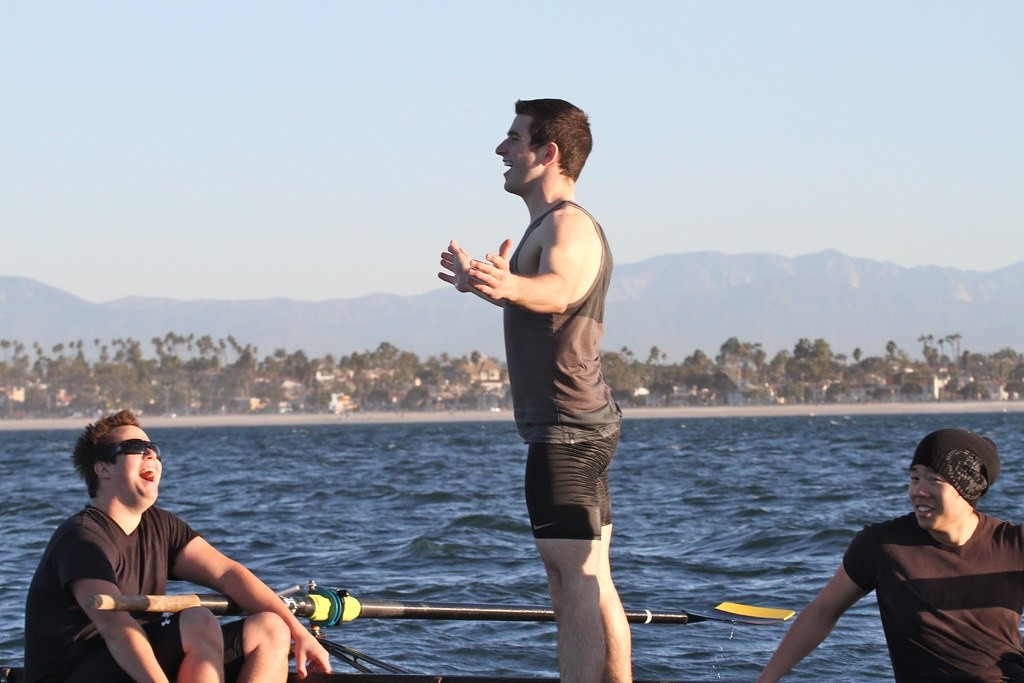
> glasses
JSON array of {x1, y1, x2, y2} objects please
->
[{"x1": 104, "y1": 438, "x2": 161, "y2": 463}]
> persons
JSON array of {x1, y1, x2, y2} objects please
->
[
  {"x1": 754, "y1": 427, "x2": 1024, "y2": 683},
  {"x1": 22, "y1": 409, "x2": 333, "y2": 683},
  {"x1": 439, "y1": 97, "x2": 635, "y2": 683}
]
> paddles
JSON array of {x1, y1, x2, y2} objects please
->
[{"x1": 91, "y1": 581, "x2": 796, "y2": 674}]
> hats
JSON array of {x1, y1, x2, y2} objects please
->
[{"x1": 912, "y1": 427, "x2": 1000, "y2": 507}]
